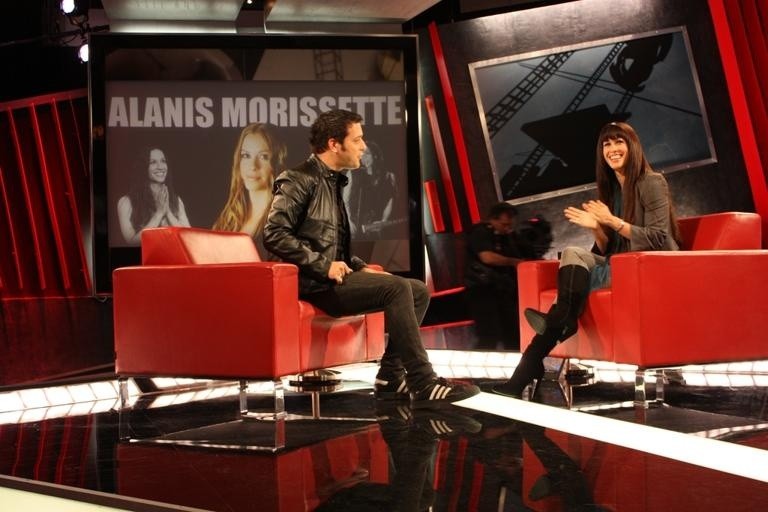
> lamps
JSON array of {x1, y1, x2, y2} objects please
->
[
  {"x1": 110, "y1": 225, "x2": 388, "y2": 455},
  {"x1": 514, "y1": 211, "x2": 766, "y2": 410}
]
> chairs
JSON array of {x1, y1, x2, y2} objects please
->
[
  {"x1": 412, "y1": 377, "x2": 481, "y2": 409},
  {"x1": 374, "y1": 399, "x2": 414, "y2": 423},
  {"x1": 413, "y1": 409, "x2": 482, "y2": 443},
  {"x1": 374, "y1": 372, "x2": 412, "y2": 400}
]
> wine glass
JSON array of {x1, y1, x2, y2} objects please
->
[{"x1": 339, "y1": 273, "x2": 343, "y2": 277}]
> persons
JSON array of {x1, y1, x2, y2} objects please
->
[
  {"x1": 117, "y1": 144, "x2": 191, "y2": 246},
  {"x1": 341, "y1": 140, "x2": 399, "y2": 241},
  {"x1": 209, "y1": 123, "x2": 302, "y2": 262},
  {"x1": 491, "y1": 123, "x2": 680, "y2": 400},
  {"x1": 463, "y1": 203, "x2": 525, "y2": 349},
  {"x1": 263, "y1": 109, "x2": 481, "y2": 409}
]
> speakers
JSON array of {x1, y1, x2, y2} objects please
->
[
  {"x1": 524, "y1": 264, "x2": 590, "y2": 343},
  {"x1": 491, "y1": 303, "x2": 557, "y2": 400}
]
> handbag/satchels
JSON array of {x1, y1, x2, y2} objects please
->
[{"x1": 616, "y1": 218, "x2": 625, "y2": 232}]
